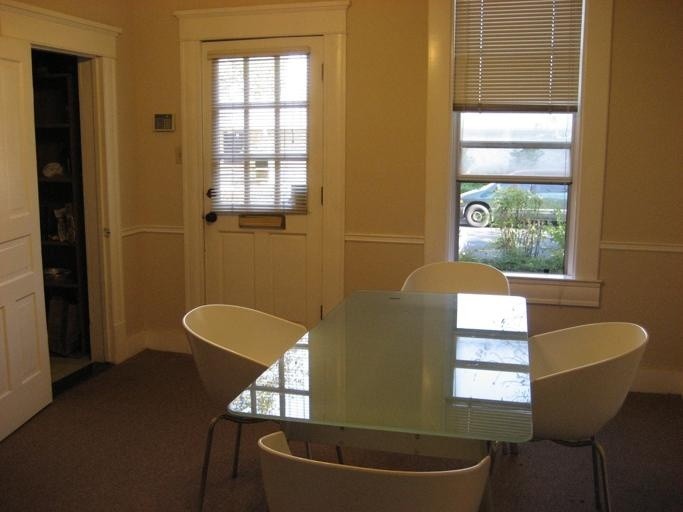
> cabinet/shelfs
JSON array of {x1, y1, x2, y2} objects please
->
[{"x1": 33, "y1": 72, "x2": 88, "y2": 358}]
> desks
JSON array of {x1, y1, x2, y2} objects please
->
[{"x1": 224, "y1": 286, "x2": 538, "y2": 452}]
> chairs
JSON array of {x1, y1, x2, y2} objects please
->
[
  {"x1": 180, "y1": 302, "x2": 347, "y2": 512},
  {"x1": 500, "y1": 320, "x2": 651, "y2": 512},
  {"x1": 391, "y1": 258, "x2": 515, "y2": 440},
  {"x1": 254, "y1": 429, "x2": 495, "y2": 511}
]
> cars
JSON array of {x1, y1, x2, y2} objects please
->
[{"x1": 460, "y1": 169, "x2": 568, "y2": 227}]
